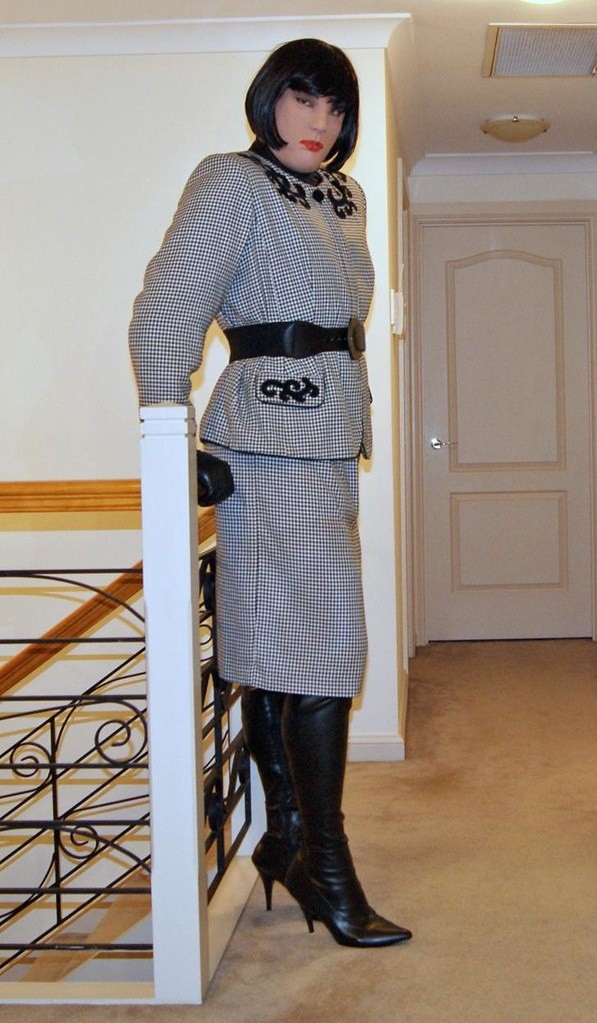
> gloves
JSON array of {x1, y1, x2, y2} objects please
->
[{"x1": 195, "y1": 450, "x2": 236, "y2": 507}]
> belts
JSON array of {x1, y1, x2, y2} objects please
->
[{"x1": 223, "y1": 317, "x2": 366, "y2": 363}]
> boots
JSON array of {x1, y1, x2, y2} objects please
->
[
  {"x1": 288, "y1": 694, "x2": 412, "y2": 948},
  {"x1": 240, "y1": 686, "x2": 304, "y2": 912}
]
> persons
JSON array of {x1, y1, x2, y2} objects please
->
[{"x1": 127, "y1": 40, "x2": 410, "y2": 948}]
